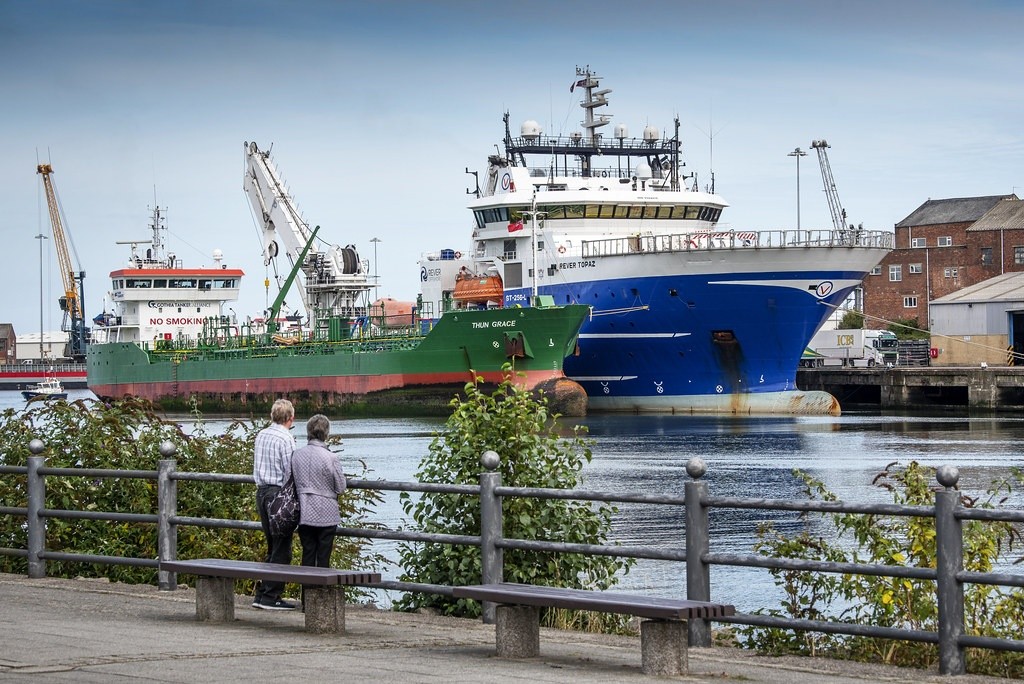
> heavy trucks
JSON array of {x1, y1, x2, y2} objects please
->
[{"x1": 799, "y1": 330, "x2": 900, "y2": 368}]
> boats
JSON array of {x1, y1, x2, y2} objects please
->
[
  {"x1": 453, "y1": 271, "x2": 504, "y2": 304},
  {"x1": 86, "y1": 183, "x2": 595, "y2": 421},
  {"x1": 21, "y1": 373, "x2": 68, "y2": 402},
  {"x1": 419, "y1": 63, "x2": 895, "y2": 416}
]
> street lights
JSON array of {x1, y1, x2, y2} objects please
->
[
  {"x1": 786, "y1": 147, "x2": 809, "y2": 246},
  {"x1": 35, "y1": 234, "x2": 48, "y2": 364},
  {"x1": 368, "y1": 237, "x2": 382, "y2": 302}
]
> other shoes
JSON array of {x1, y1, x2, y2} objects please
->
[
  {"x1": 252, "y1": 595, "x2": 261, "y2": 608},
  {"x1": 259, "y1": 595, "x2": 295, "y2": 611},
  {"x1": 302, "y1": 604, "x2": 305, "y2": 613}
]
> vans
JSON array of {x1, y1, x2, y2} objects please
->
[{"x1": 22, "y1": 360, "x2": 33, "y2": 364}]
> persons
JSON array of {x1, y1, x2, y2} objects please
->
[
  {"x1": 251, "y1": 398, "x2": 297, "y2": 610},
  {"x1": 291, "y1": 414, "x2": 346, "y2": 613}
]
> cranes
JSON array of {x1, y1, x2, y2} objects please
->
[
  {"x1": 35, "y1": 144, "x2": 83, "y2": 323},
  {"x1": 243, "y1": 141, "x2": 381, "y2": 340}
]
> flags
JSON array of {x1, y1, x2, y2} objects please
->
[{"x1": 507, "y1": 220, "x2": 523, "y2": 232}]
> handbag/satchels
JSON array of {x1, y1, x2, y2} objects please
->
[{"x1": 265, "y1": 451, "x2": 300, "y2": 538}]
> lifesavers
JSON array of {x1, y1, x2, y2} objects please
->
[
  {"x1": 602, "y1": 170, "x2": 607, "y2": 177},
  {"x1": 455, "y1": 252, "x2": 461, "y2": 259},
  {"x1": 558, "y1": 245, "x2": 566, "y2": 254}
]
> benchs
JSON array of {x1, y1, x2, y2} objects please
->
[
  {"x1": 453, "y1": 581, "x2": 736, "y2": 678},
  {"x1": 160, "y1": 559, "x2": 382, "y2": 635}
]
